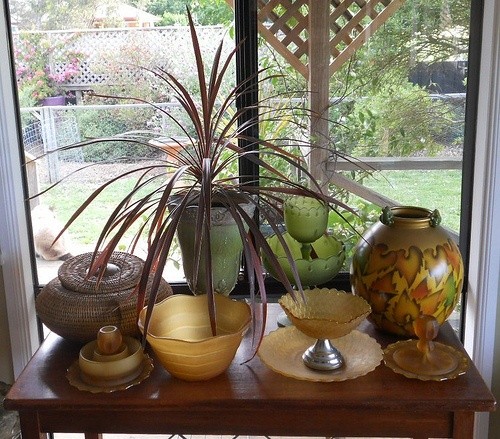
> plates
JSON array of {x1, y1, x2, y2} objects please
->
[
  {"x1": 384, "y1": 339, "x2": 469, "y2": 381},
  {"x1": 66, "y1": 354, "x2": 154, "y2": 394},
  {"x1": 257, "y1": 326, "x2": 382, "y2": 383}
]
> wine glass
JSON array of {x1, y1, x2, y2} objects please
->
[{"x1": 283, "y1": 196, "x2": 330, "y2": 258}]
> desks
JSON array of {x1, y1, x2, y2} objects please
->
[
  {"x1": 149, "y1": 135, "x2": 196, "y2": 173},
  {"x1": 3, "y1": 300, "x2": 497, "y2": 439}
]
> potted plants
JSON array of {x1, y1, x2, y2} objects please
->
[{"x1": 19, "y1": 7, "x2": 393, "y2": 364}]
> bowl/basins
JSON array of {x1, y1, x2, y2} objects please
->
[
  {"x1": 262, "y1": 230, "x2": 346, "y2": 285},
  {"x1": 78, "y1": 335, "x2": 144, "y2": 382},
  {"x1": 93, "y1": 344, "x2": 128, "y2": 362},
  {"x1": 279, "y1": 288, "x2": 372, "y2": 370},
  {"x1": 137, "y1": 292, "x2": 252, "y2": 381}
]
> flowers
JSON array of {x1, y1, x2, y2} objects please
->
[{"x1": 13, "y1": 46, "x2": 74, "y2": 99}]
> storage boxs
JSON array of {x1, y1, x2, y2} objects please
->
[{"x1": 36, "y1": 250, "x2": 173, "y2": 346}]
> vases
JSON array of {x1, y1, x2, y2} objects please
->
[{"x1": 42, "y1": 96, "x2": 68, "y2": 123}]
